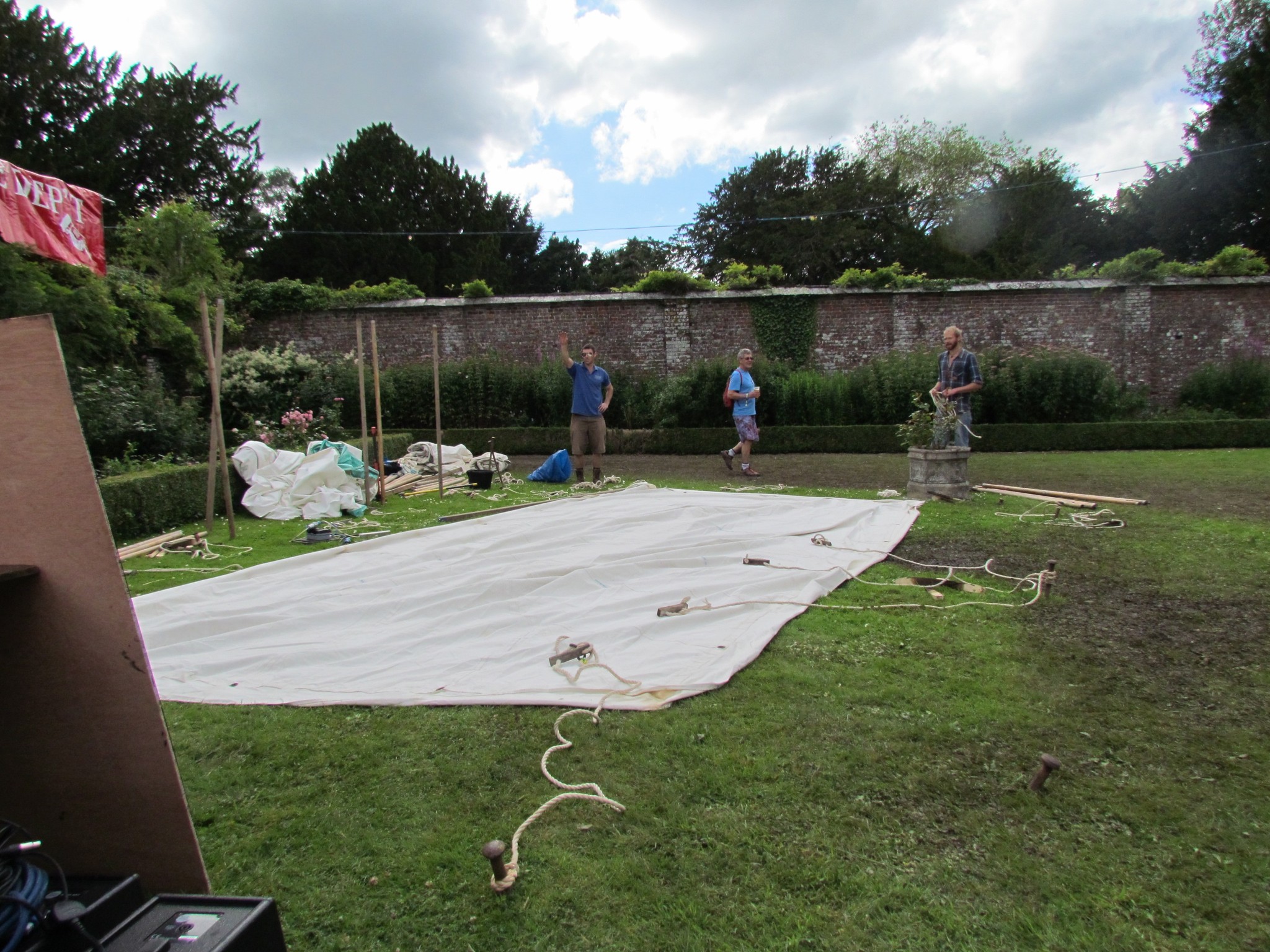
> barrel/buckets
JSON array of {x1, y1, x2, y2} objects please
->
[{"x1": 466, "y1": 470, "x2": 493, "y2": 489}]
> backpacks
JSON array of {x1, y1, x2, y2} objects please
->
[{"x1": 723, "y1": 369, "x2": 742, "y2": 408}]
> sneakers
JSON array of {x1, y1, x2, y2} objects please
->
[
  {"x1": 721, "y1": 450, "x2": 734, "y2": 471},
  {"x1": 741, "y1": 467, "x2": 760, "y2": 477}
]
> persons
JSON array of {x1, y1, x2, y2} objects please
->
[
  {"x1": 721, "y1": 348, "x2": 760, "y2": 476},
  {"x1": 929, "y1": 326, "x2": 982, "y2": 447},
  {"x1": 558, "y1": 331, "x2": 613, "y2": 488}
]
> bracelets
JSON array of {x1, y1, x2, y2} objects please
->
[{"x1": 746, "y1": 394, "x2": 748, "y2": 398}]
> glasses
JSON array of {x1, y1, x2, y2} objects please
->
[
  {"x1": 581, "y1": 352, "x2": 593, "y2": 357},
  {"x1": 742, "y1": 357, "x2": 754, "y2": 361}
]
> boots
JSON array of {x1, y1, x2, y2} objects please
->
[
  {"x1": 592, "y1": 468, "x2": 601, "y2": 486},
  {"x1": 575, "y1": 468, "x2": 585, "y2": 485}
]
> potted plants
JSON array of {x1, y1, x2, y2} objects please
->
[{"x1": 896, "y1": 390, "x2": 971, "y2": 499}]
